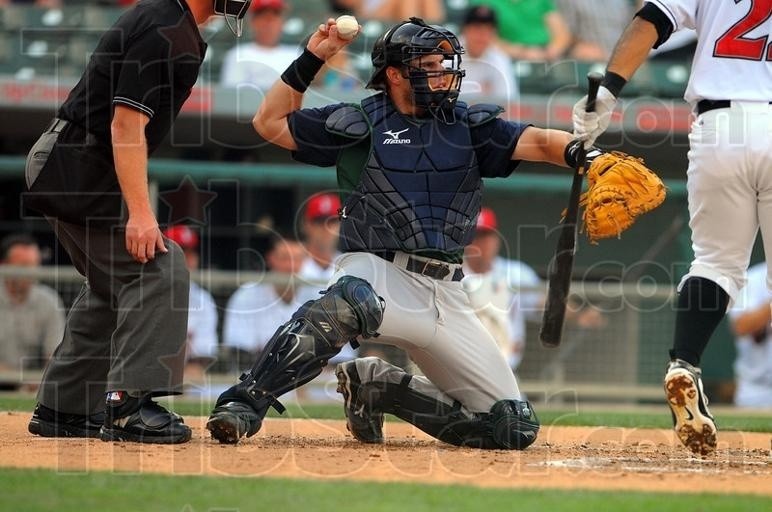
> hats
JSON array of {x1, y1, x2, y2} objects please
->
[
  {"x1": 478, "y1": 208, "x2": 497, "y2": 232},
  {"x1": 249, "y1": 0, "x2": 283, "y2": 13},
  {"x1": 306, "y1": 195, "x2": 341, "y2": 219},
  {"x1": 162, "y1": 224, "x2": 198, "y2": 246},
  {"x1": 466, "y1": 7, "x2": 495, "y2": 24}
]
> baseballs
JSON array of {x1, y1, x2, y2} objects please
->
[{"x1": 334, "y1": 15, "x2": 360, "y2": 40}]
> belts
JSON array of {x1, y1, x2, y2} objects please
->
[
  {"x1": 696, "y1": 100, "x2": 730, "y2": 114},
  {"x1": 377, "y1": 251, "x2": 464, "y2": 281},
  {"x1": 47, "y1": 118, "x2": 68, "y2": 133}
]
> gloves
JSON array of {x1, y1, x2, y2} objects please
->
[{"x1": 572, "y1": 70, "x2": 627, "y2": 150}]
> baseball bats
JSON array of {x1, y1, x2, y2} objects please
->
[{"x1": 541, "y1": 72, "x2": 604, "y2": 348}]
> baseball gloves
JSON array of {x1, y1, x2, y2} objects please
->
[{"x1": 586, "y1": 153, "x2": 667, "y2": 238}]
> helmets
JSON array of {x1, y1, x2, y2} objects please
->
[
  {"x1": 213, "y1": 0, "x2": 252, "y2": 38},
  {"x1": 365, "y1": 16, "x2": 466, "y2": 125}
]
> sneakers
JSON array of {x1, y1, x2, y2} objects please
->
[
  {"x1": 28, "y1": 402, "x2": 108, "y2": 438},
  {"x1": 101, "y1": 397, "x2": 191, "y2": 444},
  {"x1": 336, "y1": 356, "x2": 384, "y2": 443},
  {"x1": 663, "y1": 358, "x2": 718, "y2": 454},
  {"x1": 206, "y1": 401, "x2": 261, "y2": 443}
]
